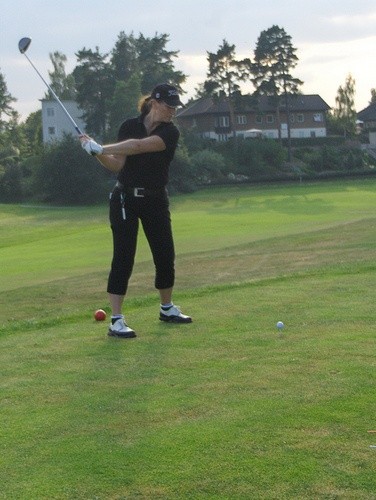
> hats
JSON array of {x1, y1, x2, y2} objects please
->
[{"x1": 151, "y1": 84, "x2": 185, "y2": 109}]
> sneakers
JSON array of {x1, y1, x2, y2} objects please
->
[
  {"x1": 108, "y1": 317, "x2": 136, "y2": 338},
  {"x1": 159, "y1": 304, "x2": 192, "y2": 323}
]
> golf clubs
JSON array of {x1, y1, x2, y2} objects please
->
[{"x1": 19, "y1": 37, "x2": 96, "y2": 156}]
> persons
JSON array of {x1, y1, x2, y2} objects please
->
[{"x1": 78, "y1": 84, "x2": 192, "y2": 336}]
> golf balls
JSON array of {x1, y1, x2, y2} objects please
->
[{"x1": 276, "y1": 321, "x2": 284, "y2": 327}]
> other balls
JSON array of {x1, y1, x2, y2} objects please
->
[{"x1": 95, "y1": 310, "x2": 106, "y2": 320}]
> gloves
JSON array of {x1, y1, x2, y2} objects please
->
[{"x1": 80, "y1": 139, "x2": 103, "y2": 156}]
